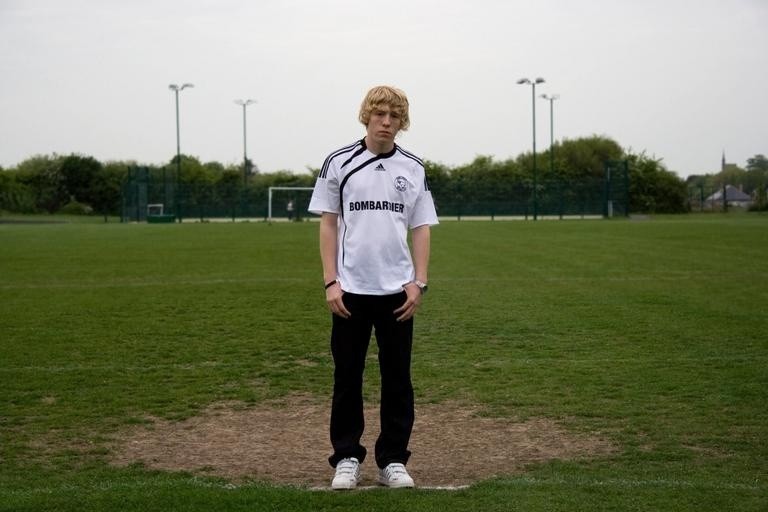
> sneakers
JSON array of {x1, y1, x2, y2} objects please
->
[
  {"x1": 332, "y1": 457, "x2": 361, "y2": 489},
  {"x1": 377, "y1": 463, "x2": 415, "y2": 488}
]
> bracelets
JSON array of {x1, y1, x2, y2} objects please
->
[{"x1": 324, "y1": 279, "x2": 338, "y2": 289}]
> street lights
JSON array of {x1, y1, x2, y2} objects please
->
[
  {"x1": 516, "y1": 77, "x2": 562, "y2": 221},
  {"x1": 234, "y1": 97, "x2": 257, "y2": 216},
  {"x1": 168, "y1": 82, "x2": 195, "y2": 224}
]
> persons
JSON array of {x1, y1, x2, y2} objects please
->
[
  {"x1": 286, "y1": 199, "x2": 293, "y2": 220},
  {"x1": 307, "y1": 86, "x2": 440, "y2": 490}
]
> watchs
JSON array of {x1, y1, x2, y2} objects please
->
[{"x1": 414, "y1": 280, "x2": 428, "y2": 293}]
